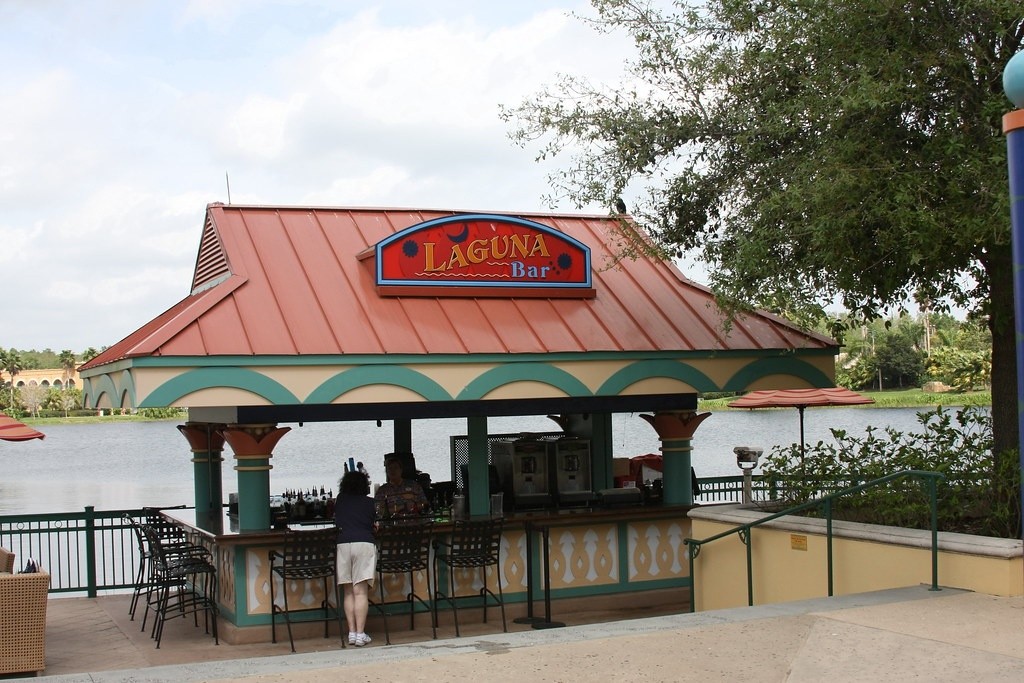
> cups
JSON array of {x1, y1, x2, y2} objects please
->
[{"x1": 492, "y1": 495, "x2": 503, "y2": 514}]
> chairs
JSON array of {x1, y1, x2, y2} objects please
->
[
  {"x1": 125, "y1": 511, "x2": 221, "y2": 650},
  {"x1": 268, "y1": 526, "x2": 345, "y2": 653},
  {"x1": 0, "y1": 547, "x2": 50, "y2": 679},
  {"x1": 432, "y1": 516, "x2": 507, "y2": 637},
  {"x1": 368, "y1": 520, "x2": 437, "y2": 646}
]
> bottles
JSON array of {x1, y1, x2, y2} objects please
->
[
  {"x1": 392, "y1": 503, "x2": 399, "y2": 525},
  {"x1": 420, "y1": 504, "x2": 426, "y2": 521},
  {"x1": 428, "y1": 504, "x2": 433, "y2": 521},
  {"x1": 403, "y1": 502, "x2": 409, "y2": 524},
  {"x1": 435, "y1": 492, "x2": 442, "y2": 522},
  {"x1": 282, "y1": 486, "x2": 336, "y2": 520},
  {"x1": 441, "y1": 491, "x2": 451, "y2": 522},
  {"x1": 381, "y1": 497, "x2": 390, "y2": 523}
]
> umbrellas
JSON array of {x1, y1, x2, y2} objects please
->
[{"x1": 726, "y1": 386, "x2": 875, "y2": 498}]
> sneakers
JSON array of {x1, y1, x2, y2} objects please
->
[
  {"x1": 349, "y1": 632, "x2": 357, "y2": 644},
  {"x1": 355, "y1": 633, "x2": 372, "y2": 646}
]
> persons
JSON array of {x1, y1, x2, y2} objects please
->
[
  {"x1": 333, "y1": 472, "x2": 378, "y2": 646},
  {"x1": 375, "y1": 458, "x2": 429, "y2": 518}
]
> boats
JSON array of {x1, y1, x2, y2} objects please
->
[{"x1": 0, "y1": 412, "x2": 46, "y2": 441}]
